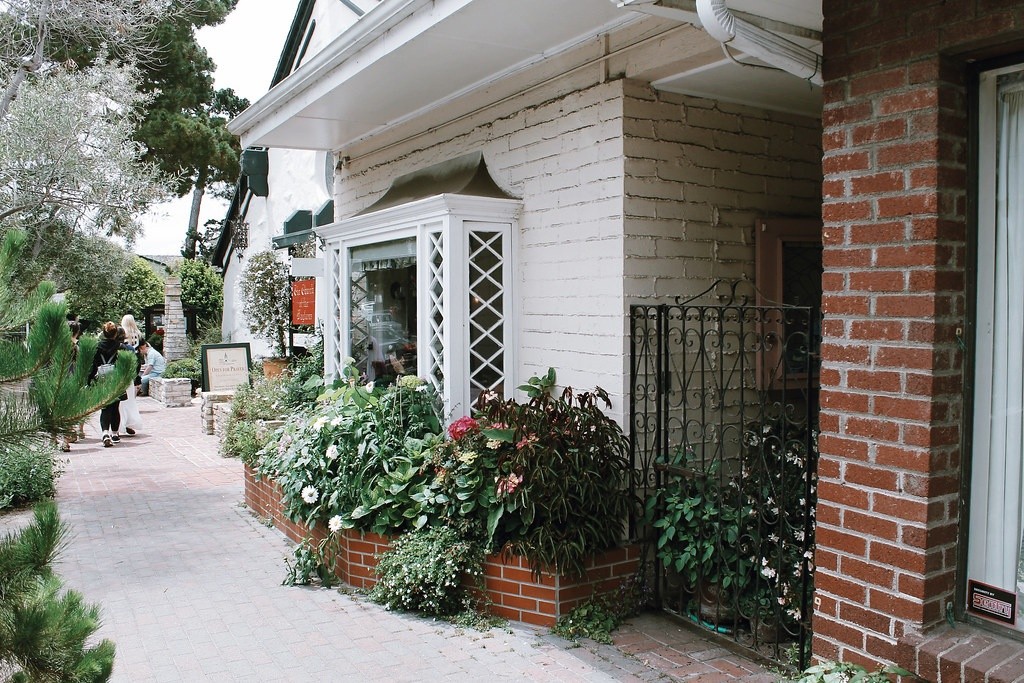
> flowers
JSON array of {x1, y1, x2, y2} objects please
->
[
  {"x1": 650, "y1": 426, "x2": 817, "y2": 615},
  {"x1": 232, "y1": 249, "x2": 294, "y2": 358},
  {"x1": 251, "y1": 368, "x2": 629, "y2": 618}
]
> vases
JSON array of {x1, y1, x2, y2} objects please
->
[
  {"x1": 747, "y1": 603, "x2": 788, "y2": 643},
  {"x1": 263, "y1": 360, "x2": 291, "y2": 377},
  {"x1": 696, "y1": 566, "x2": 741, "y2": 625}
]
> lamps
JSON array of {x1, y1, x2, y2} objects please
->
[{"x1": 229, "y1": 175, "x2": 248, "y2": 261}]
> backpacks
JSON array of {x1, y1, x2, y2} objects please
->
[
  {"x1": 95, "y1": 364, "x2": 115, "y2": 380},
  {"x1": 69, "y1": 341, "x2": 79, "y2": 373}
]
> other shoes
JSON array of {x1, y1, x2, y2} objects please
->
[
  {"x1": 126, "y1": 427, "x2": 136, "y2": 434},
  {"x1": 110, "y1": 432, "x2": 119, "y2": 437}
]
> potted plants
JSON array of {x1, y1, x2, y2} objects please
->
[{"x1": 163, "y1": 359, "x2": 202, "y2": 397}]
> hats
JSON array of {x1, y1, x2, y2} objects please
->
[{"x1": 135, "y1": 338, "x2": 146, "y2": 350}]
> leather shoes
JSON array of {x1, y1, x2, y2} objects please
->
[{"x1": 139, "y1": 393, "x2": 149, "y2": 397}]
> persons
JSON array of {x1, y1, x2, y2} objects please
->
[{"x1": 50, "y1": 314, "x2": 165, "y2": 452}]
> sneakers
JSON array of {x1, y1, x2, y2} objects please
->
[
  {"x1": 103, "y1": 434, "x2": 114, "y2": 447},
  {"x1": 77, "y1": 430, "x2": 85, "y2": 439},
  {"x1": 111, "y1": 434, "x2": 120, "y2": 443},
  {"x1": 62, "y1": 443, "x2": 71, "y2": 452}
]
